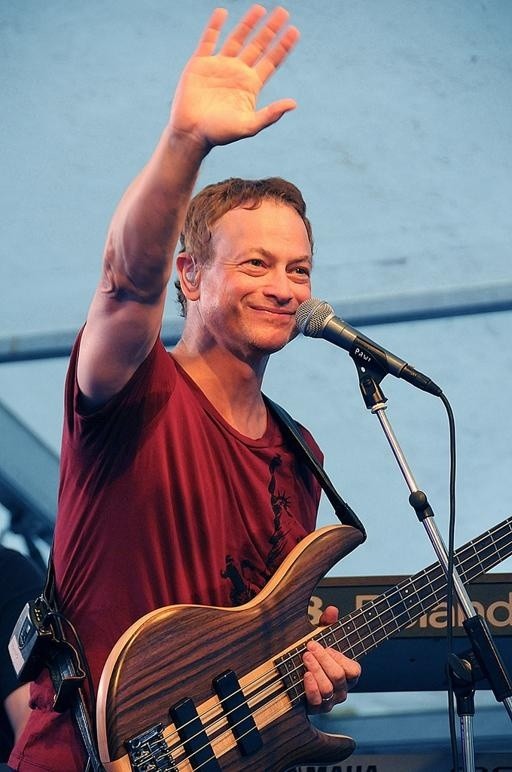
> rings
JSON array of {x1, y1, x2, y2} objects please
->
[{"x1": 324, "y1": 694, "x2": 335, "y2": 702}]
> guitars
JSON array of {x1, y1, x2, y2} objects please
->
[{"x1": 95, "y1": 516, "x2": 510, "y2": 767}]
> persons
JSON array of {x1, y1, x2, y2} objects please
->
[
  {"x1": 6, "y1": 4, "x2": 364, "y2": 772},
  {"x1": 0, "y1": 545, "x2": 48, "y2": 772}
]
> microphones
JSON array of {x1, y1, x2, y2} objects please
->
[{"x1": 294, "y1": 296, "x2": 442, "y2": 403}]
